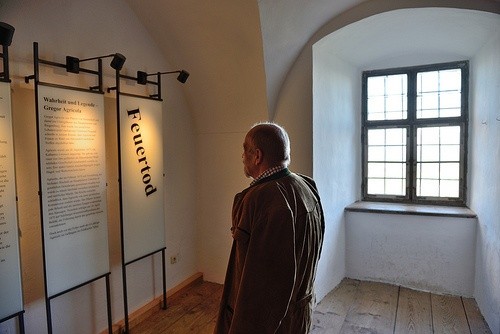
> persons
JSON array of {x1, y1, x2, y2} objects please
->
[{"x1": 213, "y1": 118, "x2": 326, "y2": 334}]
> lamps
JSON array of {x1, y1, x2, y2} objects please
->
[
  {"x1": 64, "y1": 53, "x2": 126, "y2": 75},
  {"x1": 137, "y1": 70, "x2": 190, "y2": 85}
]
